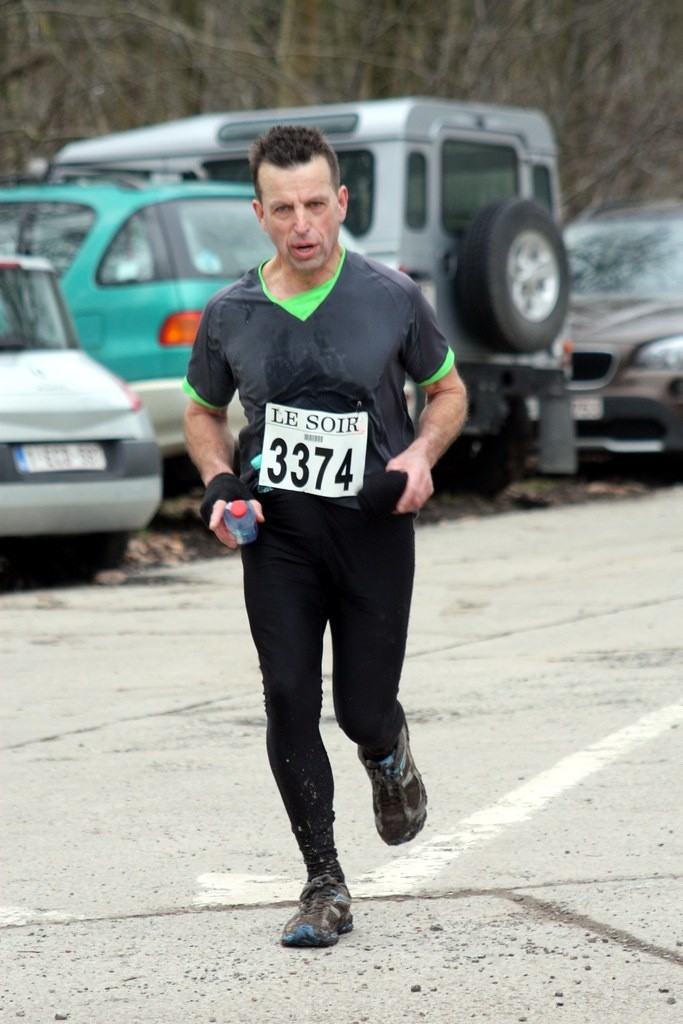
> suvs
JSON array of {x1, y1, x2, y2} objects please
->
[{"x1": 18, "y1": 95, "x2": 582, "y2": 501}]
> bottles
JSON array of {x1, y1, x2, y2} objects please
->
[{"x1": 223, "y1": 499, "x2": 259, "y2": 545}]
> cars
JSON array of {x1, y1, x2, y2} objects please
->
[
  {"x1": 2, "y1": 181, "x2": 371, "y2": 499},
  {"x1": 1, "y1": 256, "x2": 162, "y2": 574},
  {"x1": 553, "y1": 202, "x2": 682, "y2": 483}
]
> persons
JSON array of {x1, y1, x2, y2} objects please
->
[{"x1": 183, "y1": 125, "x2": 467, "y2": 948}]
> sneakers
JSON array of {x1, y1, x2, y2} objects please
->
[
  {"x1": 356, "y1": 720, "x2": 428, "y2": 844},
  {"x1": 282, "y1": 875, "x2": 352, "y2": 947}
]
